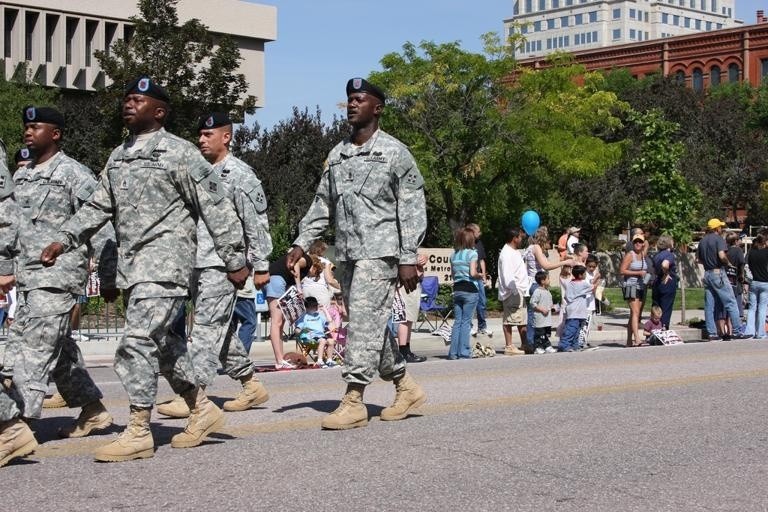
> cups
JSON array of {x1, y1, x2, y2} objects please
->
[{"x1": 633, "y1": 240, "x2": 643, "y2": 244}]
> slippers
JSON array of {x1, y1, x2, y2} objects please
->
[{"x1": 634, "y1": 342, "x2": 650, "y2": 346}]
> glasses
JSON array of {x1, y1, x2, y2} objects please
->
[{"x1": 633, "y1": 240, "x2": 643, "y2": 244}]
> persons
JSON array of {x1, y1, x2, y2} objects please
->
[
  {"x1": 8, "y1": 105, "x2": 120, "y2": 438},
  {"x1": 0, "y1": 148, "x2": 68, "y2": 407},
  {"x1": 40, "y1": 75, "x2": 250, "y2": 462},
  {"x1": 0, "y1": 140, "x2": 38, "y2": 467},
  {"x1": 157, "y1": 113, "x2": 269, "y2": 418},
  {"x1": 448, "y1": 217, "x2": 767, "y2": 360},
  {"x1": 231, "y1": 240, "x2": 427, "y2": 372},
  {"x1": 287, "y1": 77, "x2": 428, "y2": 430}
]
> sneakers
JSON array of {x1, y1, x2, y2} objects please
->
[
  {"x1": 709, "y1": 333, "x2": 767, "y2": 342},
  {"x1": 504, "y1": 344, "x2": 599, "y2": 354},
  {"x1": 449, "y1": 354, "x2": 479, "y2": 360},
  {"x1": 471, "y1": 327, "x2": 493, "y2": 336},
  {"x1": 274, "y1": 359, "x2": 297, "y2": 370},
  {"x1": 315, "y1": 360, "x2": 338, "y2": 368},
  {"x1": 404, "y1": 352, "x2": 427, "y2": 362}
]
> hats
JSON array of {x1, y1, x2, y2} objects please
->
[
  {"x1": 707, "y1": 218, "x2": 726, "y2": 231},
  {"x1": 124, "y1": 77, "x2": 171, "y2": 103},
  {"x1": 632, "y1": 234, "x2": 645, "y2": 243},
  {"x1": 15, "y1": 146, "x2": 34, "y2": 163},
  {"x1": 346, "y1": 77, "x2": 385, "y2": 105},
  {"x1": 22, "y1": 105, "x2": 65, "y2": 129},
  {"x1": 569, "y1": 226, "x2": 581, "y2": 234},
  {"x1": 198, "y1": 116, "x2": 232, "y2": 133}
]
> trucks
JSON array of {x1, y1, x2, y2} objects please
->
[{"x1": 522, "y1": 211, "x2": 540, "y2": 237}]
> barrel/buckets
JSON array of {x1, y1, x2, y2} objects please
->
[{"x1": 634, "y1": 342, "x2": 650, "y2": 346}]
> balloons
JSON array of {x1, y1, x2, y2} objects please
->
[{"x1": 522, "y1": 211, "x2": 540, "y2": 237}]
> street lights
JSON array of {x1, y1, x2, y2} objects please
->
[
  {"x1": 621, "y1": 284, "x2": 636, "y2": 300},
  {"x1": 472, "y1": 345, "x2": 497, "y2": 358}
]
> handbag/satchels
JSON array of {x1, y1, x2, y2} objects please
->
[
  {"x1": 621, "y1": 284, "x2": 636, "y2": 300},
  {"x1": 472, "y1": 345, "x2": 497, "y2": 358}
]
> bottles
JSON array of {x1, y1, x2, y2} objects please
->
[
  {"x1": 15, "y1": 146, "x2": 34, "y2": 163},
  {"x1": 124, "y1": 77, "x2": 171, "y2": 103},
  {"x1": 632, "y1": 234, "x2": 645, "y2": 243},
  {"x1": 707, "y1": 218, "x2": 726, "y2": 231},
  {"x1": 346, "y1": 77, "x2": 385, "y2": 105},
  {"x1": 22, "y1": 105, "x2": 65, "y2": 129},
  {"x1": 198, "y1": 116, "x2": 232, "y2": 133},
  {"x1": 569, "y1": 226, "x2": 581, "y2": 234}
]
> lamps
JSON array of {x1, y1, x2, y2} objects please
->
[{"x1": 417, "y1": 276, "x2": 453, "y2": 333}]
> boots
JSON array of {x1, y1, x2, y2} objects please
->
[
  {"x1": 42, "y1": 391, "x2": 67, "y2": 408},
  {"x1": 3, "y1": 416, "x2": 38, "y2": 465},
  {"x1": 158, "y1": 394, "x2": 190, "y2": 417},
  {"x1": 322, "y1": 383, "x2": 368, "y2": 430},
  {"x1": 59, "y1": 401, "x2": 113, "y2": 438},
  {"x1": 223, "y1": 371, "x2": 269, "y2": 411},
  {"x1": 93, "y1": 405, "x2": 154, "y2": 462},
  {"x1": 380, "y1": 370, "x2": 424, "y2": 420},
  {"x1": 171, "y1": 386, "x2": 225, "y2": 449}
]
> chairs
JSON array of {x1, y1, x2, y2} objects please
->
[
  {"x1": 404, "y1": 352, "x2": 427, "y2": 362},
  {"x1": 315, "y1": 360, "x2": 338, "y2": 368},
  {"x1": 709, "y1": 333, "x2": 767, "y2": 342},
  {"x1": 471, "y1": 327, "x2": 493, "y2": 336},
  {"x1": 274, "y1": 359, "x2": 297, "y2": 370},
  {"x1": 449, "y1": 354, "x2": 479, "y2": 360},
  {"x1": 504, "y1": 344, "x2": 599, "y2": 354}
]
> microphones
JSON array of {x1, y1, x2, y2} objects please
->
[{"x1": 291, "y1": 336, "x2": 344, "y2": 364}]
